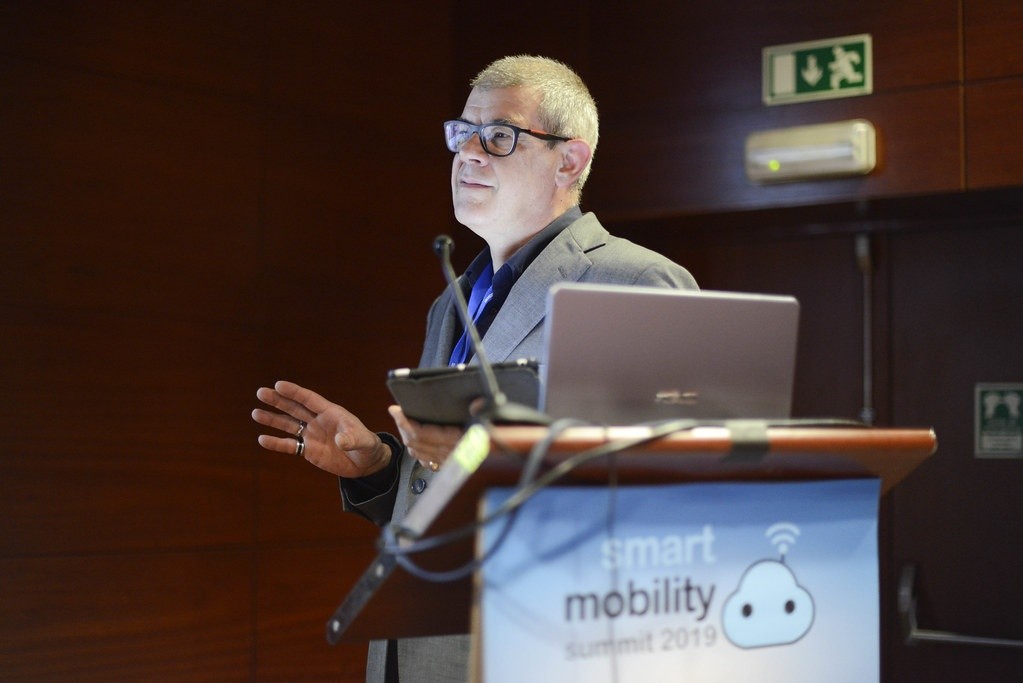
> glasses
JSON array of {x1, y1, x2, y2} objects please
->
[{"x1": 444, "y1": 119, "x2": 572, "y2": 156}]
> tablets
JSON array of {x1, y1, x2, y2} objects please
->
[{"x1": 387, "y1": 357, "x2": 539, "y2": 427}]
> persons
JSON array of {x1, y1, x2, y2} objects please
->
[{"x1": 250, "y1": 54, "x2": 702, "y2": 683}]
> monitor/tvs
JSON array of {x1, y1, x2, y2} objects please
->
[{"x1": 538, "y1": 283, "x2": 799, "y2": 424}]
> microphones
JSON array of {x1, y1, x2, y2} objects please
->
[{"x1": 433, "y1": 236, "x2": 504, "y2": 403}]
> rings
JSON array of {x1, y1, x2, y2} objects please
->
[
  {"x1": 295, "y1": 439, "x2": 305, "y2": 456},
  {"x1": 295, "y1": 421, "x2": 306, "y2": 437},
  {"x1": 429, "y1": 462, "x2": 438, "y2": 471}
]
